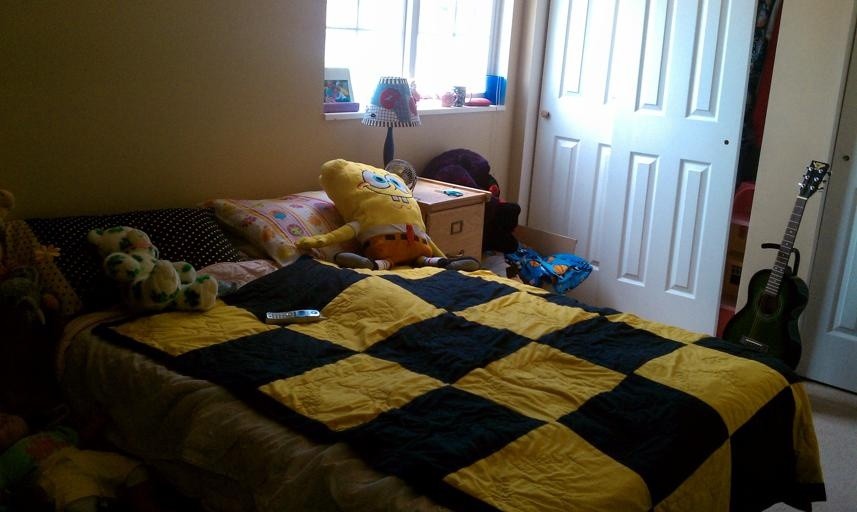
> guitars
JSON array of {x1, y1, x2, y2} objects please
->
[{"x1": 722, "y1": 161, "x2": 831, "y2": 372}]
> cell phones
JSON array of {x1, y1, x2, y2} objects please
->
[{"x1": 264, "y1": 308, "x2": 328, "y2": 326}]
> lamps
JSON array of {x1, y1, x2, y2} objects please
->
[{"x1": 361, "y1": 77, "x2": 421, "y2": 169}]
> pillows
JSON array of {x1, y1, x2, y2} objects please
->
[
  {"x1": 0, "y1": 208, "x2": 249, "y2": 316},
  {"x1": 196, "y1": 190, "x2": 361, "y2": 269}
]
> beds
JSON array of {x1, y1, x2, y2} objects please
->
[{"x1": 1, "y1": 192, "x2": 829, "y2": 512}]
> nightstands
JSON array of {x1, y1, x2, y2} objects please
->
[{"x1": 413, "y1": 177, "x2": 492, "y2": 264}]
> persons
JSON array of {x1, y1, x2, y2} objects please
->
[{"x1": 324, "y1": 81, "x2": 351, "y2": 102}]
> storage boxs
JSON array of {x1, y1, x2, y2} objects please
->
[{"x1": 514, "y1": 222, "x2": 577, "y2": 291}]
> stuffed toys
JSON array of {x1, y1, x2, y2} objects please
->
[
  {"x1": 421, "y1": 148, "x2": 521, "y2": 254},
  {"x1": 0, "y1": 264, "x2": 59, "y2": 325},
  {"x1": 87, "y1": 224, "x2": 219, "y2": 311},
  {"x1": 0, "y1": 413, "x2": 150, "y2": 512},
  {"x1": 293, "y1": 158, "x2": 480, "y2": 272}
]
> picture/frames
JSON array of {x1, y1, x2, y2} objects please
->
[{"x1": 324, "y1": 67, "x2": 355, "y2": 103}]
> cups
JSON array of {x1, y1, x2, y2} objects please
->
[
  {"x1": 442, "y1": 92, "x2": 458, "y2": 107},
  {"x1": 453, "y1": 86, "x2": 466, "y2": 106},
  {"x1": 485, "y1": 74, "x2": 504, "y2": 106}
]
